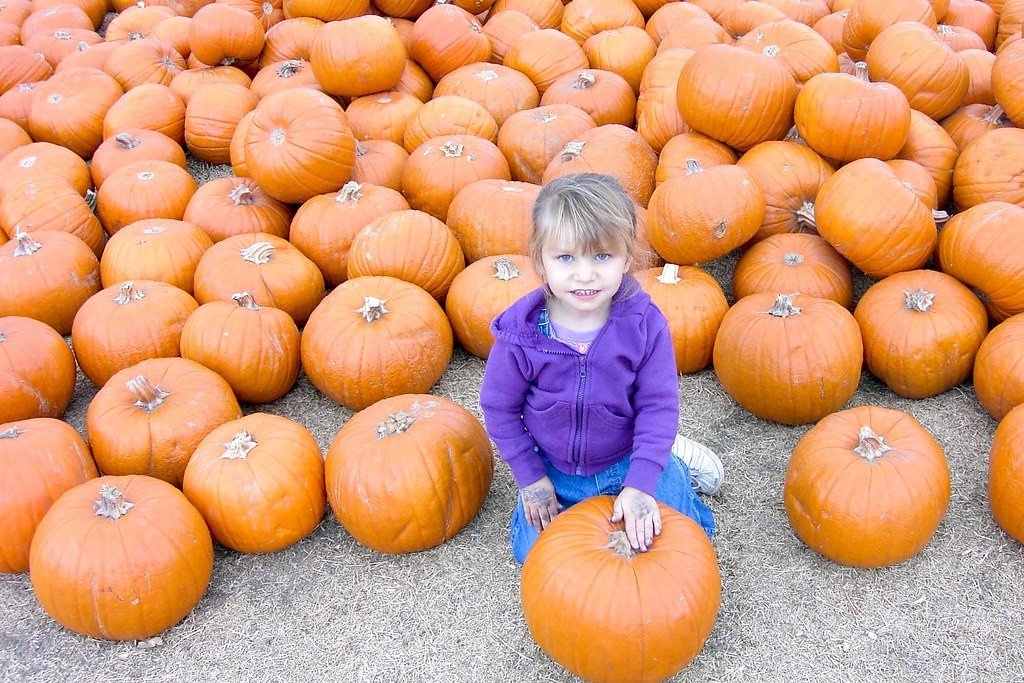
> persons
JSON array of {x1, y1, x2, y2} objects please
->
[{"x1": 480, "y1": 172, "x2": 724, "y2": 565}]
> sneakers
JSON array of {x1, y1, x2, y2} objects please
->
[{"x1": 671, "y1": 433, "x2": 725, "y2": 495}]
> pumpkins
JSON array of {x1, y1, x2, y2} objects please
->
[
  {"x1": 520, "y1": 494, "x2": 720, "y2": 683},
  {"x1": 0, "y1": 0, "x2": 1024, "y2": 639}
]
